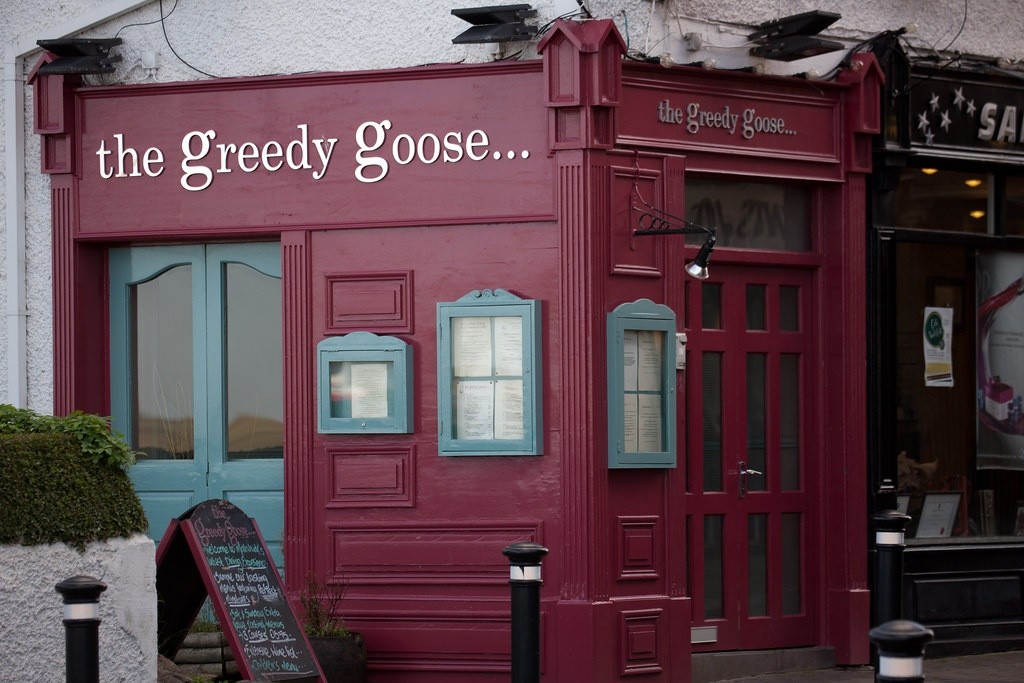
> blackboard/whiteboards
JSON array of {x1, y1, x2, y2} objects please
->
[{"x1": 178, "y1": 499, "x2": 328, "y2": 683}]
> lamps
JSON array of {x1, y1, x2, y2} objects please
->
[
  {"x1": 502, "y1": 543, "x2": 549, "y2": 587},
  {"x1": 871, "y1": 510, "x2": 912, "y2": 551},
  {"x1": 749, "y1": 9, "x2": 845, "y2": 63},
  {"x1": 451, "y1": 3, "x2": 537, "y2": 45},
  {"x1": 37, "y1": 38, "x2": 123, "y2": 74},
  {"x1": 55, "y1": 576, "x2": 107, "y2": 629},
  {"x1": 630, "y1": 147, "x2": 717, "y2": 280},
  {"x1": 869, "y1": 619, "x2": 935, "y2": 683}
]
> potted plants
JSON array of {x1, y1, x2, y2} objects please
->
[{"x1": 296, "y1": 570, "x2": 367, "y2": 683}]
